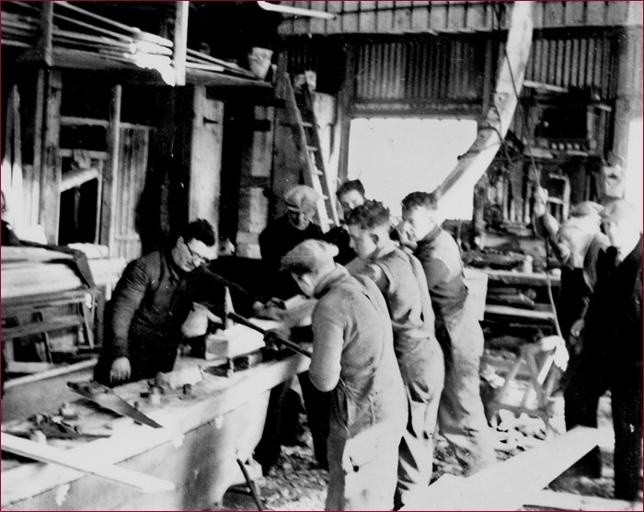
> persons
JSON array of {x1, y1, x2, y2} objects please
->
[
  {"x1": 261, "y1": 240, "x2": 408, "y2": 511},
  {"x1": 338, "y1": 200, "x2": 444, "y2": 509},
  {"x1": 530, "y1": 184, "x2": 613, "y2": 366},
  {"x1": 252, "y1": 186, "x2": 333, "y2": 473},
  {"x1": 321, "y1": 179, "x2": 403, "y2": 266},
  {"x1": 401, "y1": 191, "x2": 497, "y2": 477},
  {"x1": 0, "y1": 188, "x2": 20, "y2": 246},
  {"x1": 556, "y1": 215, "x2": 611, "y2": 339},
  {"x1": 92, "y1": 219, "x2": 290, "y2": 389},
  {"x1": 557, "y1": 195, "x2": 643, "y2": 502}
]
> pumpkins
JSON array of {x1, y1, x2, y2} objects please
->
[{"x1": 270, "y1": 71, "x2": 341, "y2": 233}]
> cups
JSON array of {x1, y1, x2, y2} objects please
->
[{"x1": 185, "y1": 241, "x2": 208, "y2": 265}]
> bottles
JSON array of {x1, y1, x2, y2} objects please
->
[
  {"x1": 280, "y1": 239, "x2": 339, "y2": 270},
  {"x1": 597, "y1": 200, "x2": 638, "y2": 222},
  {"x1": 284, "y1": 185, "x2": 320, "y2": 212},
  {"x1": 572, "y1": 201, "x2": 605, "y2": 216}
]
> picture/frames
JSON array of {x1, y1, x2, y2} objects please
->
[
  {"x1": 1, "y1": 283, "x2": 106, "y2": 353},
  {"x1": 467, "y1": 265, "x2": 561, "y2": 336}
]
